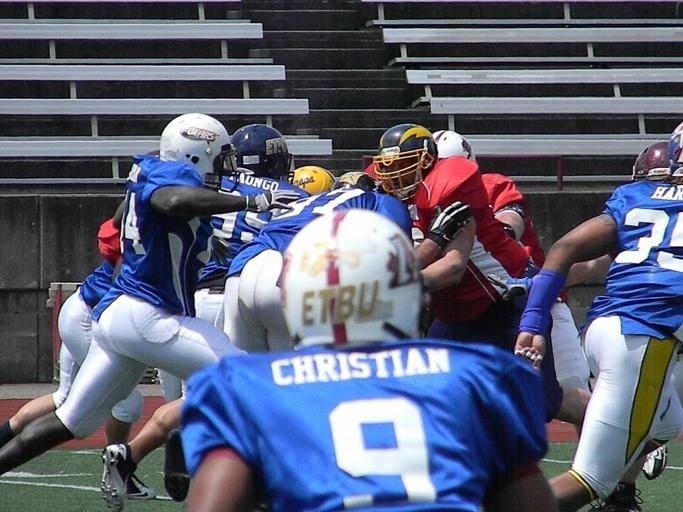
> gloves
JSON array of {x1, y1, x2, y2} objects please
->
[
  {"x1": 486, "y1": 273, "x2": 532, "y2": 300},
  {"x1": 425, "y1": 201, "x2": 473, "y2": 250},
  {"x1": 246, "y1": 189, "x2": 301, "y2": 212}
]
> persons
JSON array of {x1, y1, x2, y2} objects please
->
[{"x1": 0, "y1": 113, "x2": 682, "y2": 512}]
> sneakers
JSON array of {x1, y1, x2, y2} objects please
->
[
  {"x1": 587, "y1": 488, "x2": 644, "y2": 512},
  {"x1": 642, "y1": 445, "x2": 667, "y2": 480},
  {"x1": 97, "y1": 423, "x2": 188, "y2": 511}
]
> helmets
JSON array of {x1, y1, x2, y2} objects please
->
[
  {"x1": 281, "y1": 208, "x2": 423, "y2": 345},
  {"x1": 633, "y1": 122, "x2": 683, "y2": 181}
]
